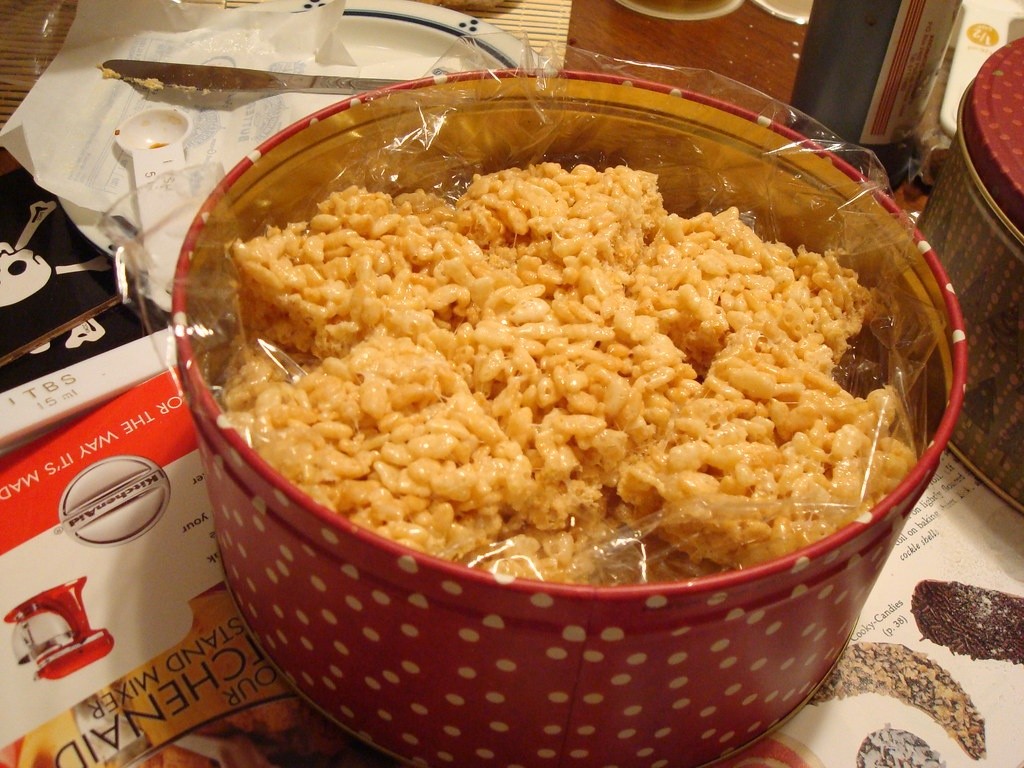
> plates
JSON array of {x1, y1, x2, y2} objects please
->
[
  {"x1": 60, "y1": 0, "x2": 561, "y2": 312},
  {"x1": 939, "y1": 0, "x2": 1024, "y2": 140}
]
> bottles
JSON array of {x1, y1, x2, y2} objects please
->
[{"x1": 783, "y1": 0, "x2": 962, "y2": 193}]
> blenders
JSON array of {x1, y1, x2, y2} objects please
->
[{"x1": 3, "y1": 576, "x2": 114, "y2": 680}]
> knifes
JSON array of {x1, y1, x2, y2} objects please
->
[{"x1": 103, "y1": 61, "x2": 407, "y2": 94}]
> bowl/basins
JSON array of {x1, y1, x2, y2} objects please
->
[{"x1": 172, "y1": 69, "x2": 969, "y2": 768}]
